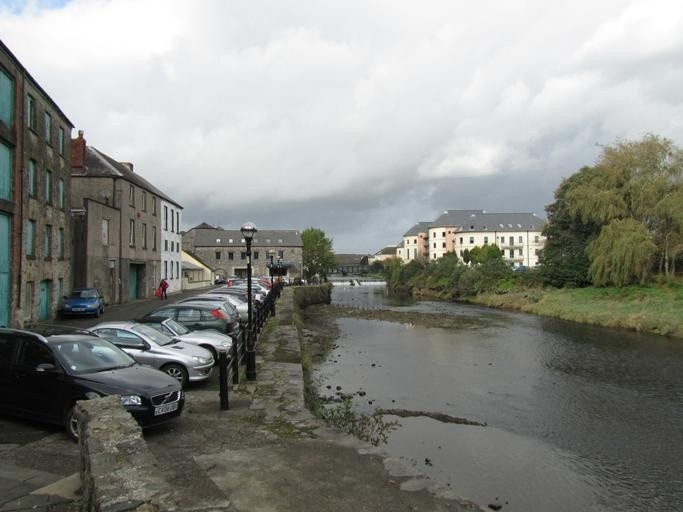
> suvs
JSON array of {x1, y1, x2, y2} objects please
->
[{"x1": 133, "y1": 301, "x2": 243, "y2": 357}]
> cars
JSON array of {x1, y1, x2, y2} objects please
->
[
  {"x1": 55, "y1": 319, "x2": 216, "y2": 389},
  {"x1": 57, "y1": 287, "x2": 105, "y2": 321},
  {"x1": 174, "y1": 275, "x2": 295, "y2": 328},
  {"x1": 0, "y1": 322, "x2": 186, "y2": 444},
  {"x1": 115, "y1": 316, "x2": 233, "y2": 367}
]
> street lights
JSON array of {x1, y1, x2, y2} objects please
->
[
  {"x1": 265, "y1": 248, "x2": 286, "y2": 316},
  {"x1": 239, "y1": 220, "x2": 260, "y2": 382}
]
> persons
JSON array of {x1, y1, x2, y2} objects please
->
[{"x1": 157, "y1": 278, "x2": 169, "y2": 300}]
